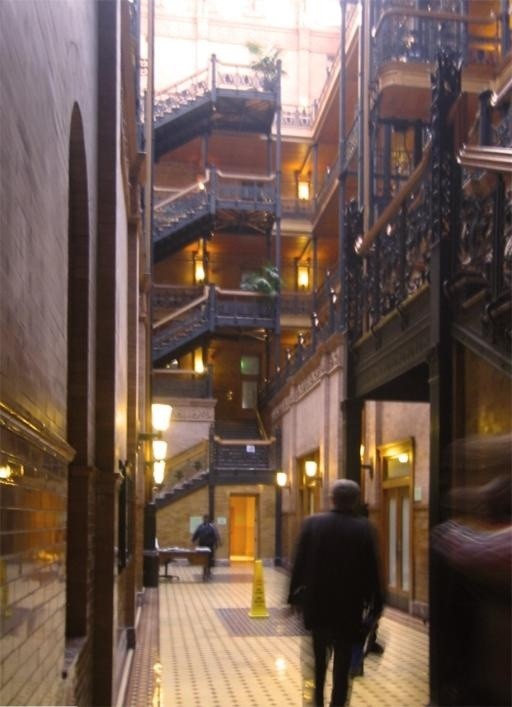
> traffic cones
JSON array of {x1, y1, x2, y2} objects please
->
[{"x1": 247, "y1": 558, "x2": 270, "y2": 618}]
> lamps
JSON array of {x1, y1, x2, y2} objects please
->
[
  {"x1": 359, "y1": 442, "x2": 375, "y2": 481},
  {"x1": 293, "y1": 254, "x2": 312, "y2": 294},
  {"x1": 292, "y1": 170, "x2": 313, "y2": 212},
  {"x1": 138, "y1": 403, "x2": 173, "y2": 486},
  {"x1": 191, "y1": 249, "x2": 206, "y2": 287},
  {"x1": 276, "y1": 459, "x2": 322, "y2": 491}
]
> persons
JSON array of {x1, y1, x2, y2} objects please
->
[
  {"x1": 192, "y1": 512, "x2": 223, "y2": 580},
  {"x1": 287, "y1": 478, "x2": 385, "y2": 705},
  {"x1": 355, "y1": 502, "x2": 384, "y2": 656}
]
